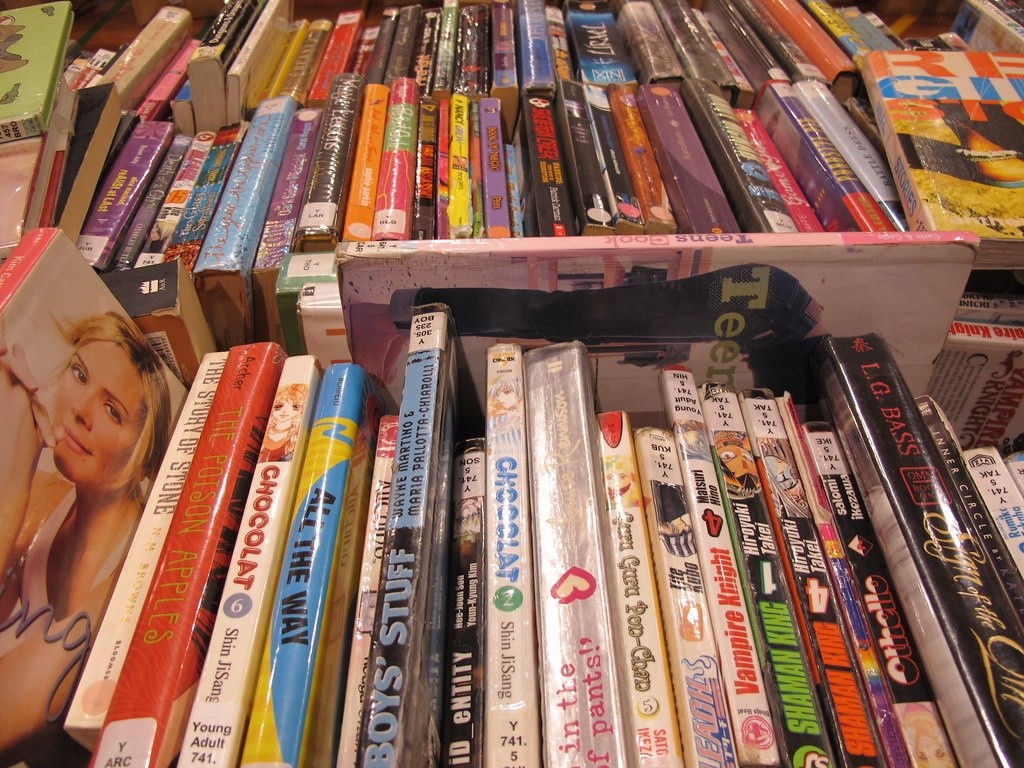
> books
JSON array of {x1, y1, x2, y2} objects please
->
[{"x1": 0, "y1": 1, "x2": 1022, "y2": 766}]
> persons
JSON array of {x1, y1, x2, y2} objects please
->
[{"x1": 0, "y1": 309, "x2": 174, "y2": 751}]
